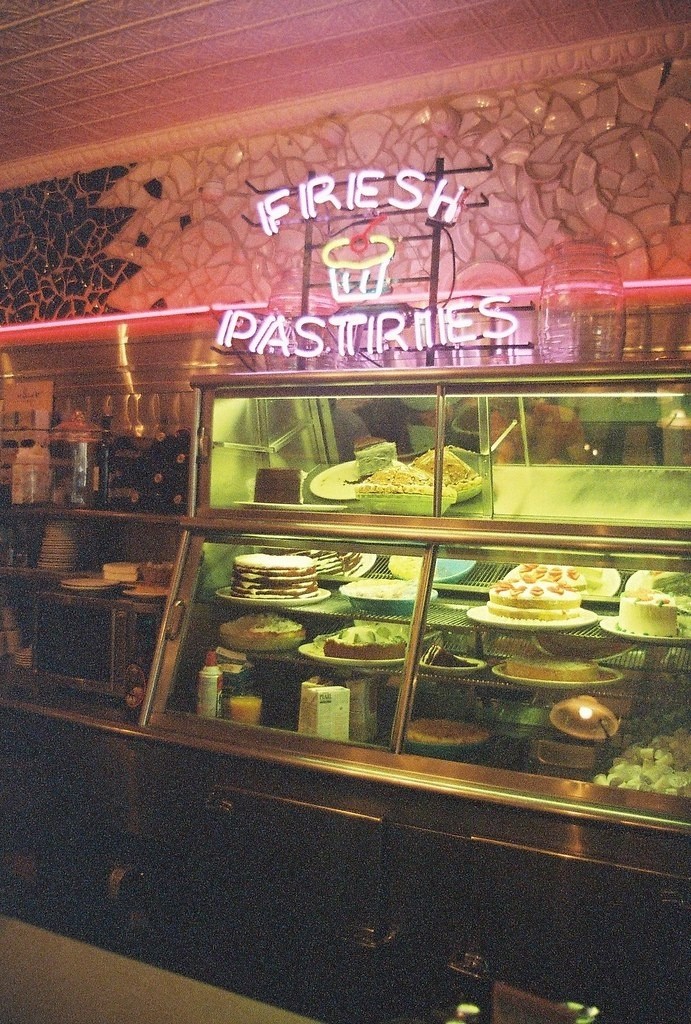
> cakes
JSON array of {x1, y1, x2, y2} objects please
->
[
  {"x1": 505, "y1": 652, "x2": 601, "y2": 683},
  {"x1": 512, "y1": 564, "x2": 589, "y2": 593},
  {"x1": 487, "y1": 576, "x2": 583, "y2": 621},
  {"x1": 254, "y1": 467, "x2": 306, "y2": 504},
  {"x1": 280, "y1": 549, "x2": 362, "y2": 577},
  {"x1": 323, "y1": 625, "x2": 406, "y2": 661},
  {"x1": 642, "y1": 571, "x2": 691, "y2": 594},
  {"x1": 353, "y1": 436, "x2": 396, "y2": 478},
  {"x1": 619, "y1": 587, "x2": 679, "y2": 637},
  {"x1": 220, "y1": 610, "x2": 307, "y2": 648},
  {"x1": 229, "y1": 553, "x2": 318, "y2": 601},
  {"x1": 411, "y1": 447, "x2": 483, "y2": 492},
  {"x1": 354, "y1": 460, "x2": 458, "y2": 497}
]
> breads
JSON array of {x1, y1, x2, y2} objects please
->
[{"x1": 404, "y1": 717, "x2": 492, "y2": 743}]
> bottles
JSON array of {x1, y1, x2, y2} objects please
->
[
  {"x1": 92, "y1": 416, "x2": 192, "y2": 513},
  {"x1": 196, "y1": 652, "x2": 223, "y2": 718}
]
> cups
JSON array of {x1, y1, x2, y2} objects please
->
[{"x1": 230, "y1": 695, "x2": 263, "y2": 726}]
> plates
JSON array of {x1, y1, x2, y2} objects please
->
[
  {"x1": 60, "y1": 579, "x2": 120, "y2": 591},
  {"x1": 625, "y1": 570, "x2": 649, "y2": 591},
  {"x1": 339, "y1": 580, "x2": 438, "y2": 614},
  {"x1": 37, "y1": 521, "x2": 78, "y2": 570},
  {"x1": 298, "y1": 642, "x2": 405, "y2": 668},
  {"x1": 467, "y1": 606, "x2": 598, "y2": 631},
  {"x1": 492, "y1": 664, "x2": 624, "y2": 689},
  {"x1": 600, "y1": 616, "x2": 690, "y2": 645},
  {"x1": 388, "y1": 555, "x2": 476, "y2": 584},
  {"x1": 15, "y1": 649, "x2": 32, "y2": 669},
  {"x1": 234, "y1": 501, "x2": 347, "y2": 513},
  {"x1": 406, "y1": 738, "x2": 496, "y2": 759},
  {"x1": 349, "y1": 553, "x2": 377, "y2": 577},
  {"x1": 310, "y1": 459, "x2": 406, "y2": 500},
  {"x1": 419, "y1": 656, "x2": 487, "y2": 676},
  {"x1": 214, "y1": 586, "x2": 332, "y2": 606},
  {"x1": 456, "y1": 485, "x2": 482, "y2": 504},
  {"x1": 124, "y1": 590, "x2": 169, "y2": 599},
  {"x1": 504, "y1": 563, "x2": 621, "y2": 597},
  {"x1": 531, "y1": 635, "x2": 637, "y2": 662},
  {"x1": 356, "y1": 493, "x2": 458, "y2": 516}
]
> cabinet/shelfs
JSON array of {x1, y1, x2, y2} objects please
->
[{"x1": 0, "y1": 375, "x2": 691, "y2": 1024}]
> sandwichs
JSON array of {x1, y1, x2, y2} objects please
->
[{"x1": 422, "y1": 643, "x2": 473, "y2": 667}]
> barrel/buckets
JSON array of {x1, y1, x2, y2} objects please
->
[
  {"x1": 48, "y1": 411, "x2": 101, "y2": 508},
  {"x1": 537, "y1": 240, "x2": 626, "y2": 363},
  {"x1": 264, "y1": 268, "x2": 339, "y2": 371},
  {"x1": 11, "y1": 448, "x2": 50, "y2": 507}
]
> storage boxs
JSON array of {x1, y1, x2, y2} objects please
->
[
  {"x1": 305, "y1": 685, "x2": 349, "y2": 740},
  {"x1": 297, "y1": 675, "x2": 333, "y2": 733},
  {"x1": 347, "y1": 670, "x2": 378, "y2": 743}
]
